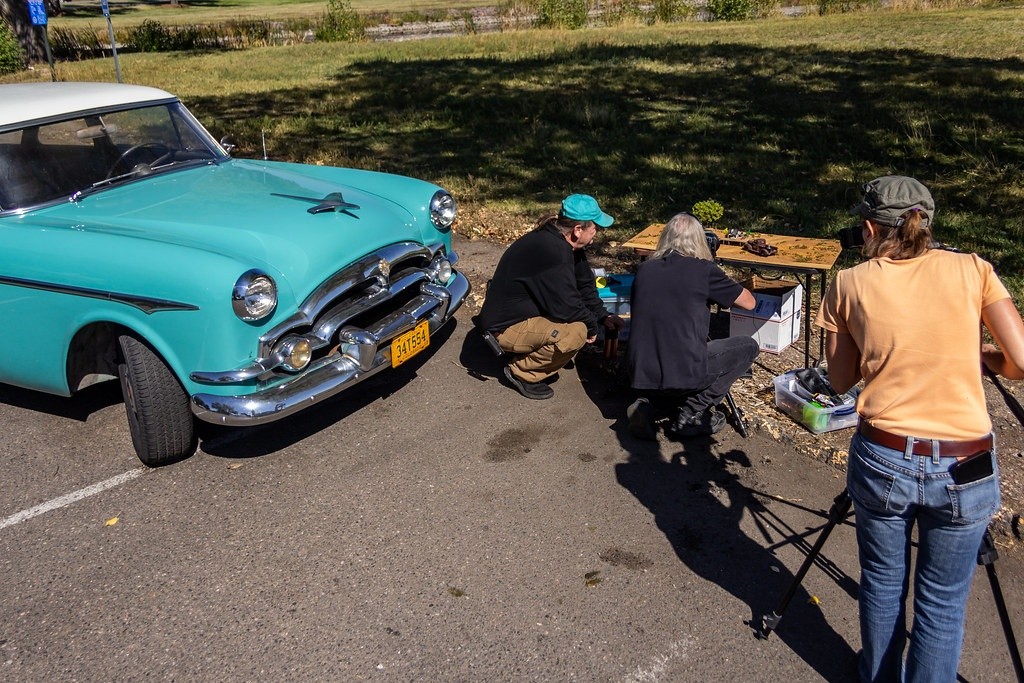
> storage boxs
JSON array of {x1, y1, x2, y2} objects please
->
[
  {"x1": 730, "y1": 276, "x2": 802, "y2": 355},
  {"x1": 772, "y1": 369, "x2": 861, "y2": 435},
  {"x1": 596, "y1": 273, "x2": 635, "y2": 341}
]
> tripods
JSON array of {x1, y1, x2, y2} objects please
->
[{"x1": 760, "y1": 363, "x2": 1024, "y2": 683}]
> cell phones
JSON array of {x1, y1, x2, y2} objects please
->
[{"x1": 954, "y1": 450, "x2": 993, "y2": 485}]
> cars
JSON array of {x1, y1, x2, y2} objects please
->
[{"x1": 0, "y1": 84, "x2": 475, "y2": 467}]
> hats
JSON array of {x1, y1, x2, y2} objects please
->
[
  {"x1": 857, "y1": 175, "x2": 935, "y2": 229},
  {"x1": 561, "y1": 194, "x2": 614, "y2": 228}
]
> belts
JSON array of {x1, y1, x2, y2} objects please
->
[{"x1": 856, "y1": 416, "x2": 993, "y2": 457}]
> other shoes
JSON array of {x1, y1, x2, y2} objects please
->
[
  {"x1": 627, "y1": 403, "x2": 655, "y2": 441},
  {"x1": 504, "y1": 367, "x2": 554, "y2": 400},
  {"x1": 671, "y1": 406, "x2": 726, "y2": 435}
]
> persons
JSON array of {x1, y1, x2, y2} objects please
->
[
  {"x1": 626, "y1": 211, "x2": 761, "y2": 444},
  {"x1": 813, "y1": 175, "x2": 1024, "y2": 683},
  {"x1": 477, "y1": 193, "x2": 625, "y2": 400}
]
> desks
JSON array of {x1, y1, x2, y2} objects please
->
[{"x1": 621, "y1": 222, "x2": 842, "y2": 369}]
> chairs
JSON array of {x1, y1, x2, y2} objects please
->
[{"x1": 0, "y1": 150, "x2": 63, "y2": 212}]
